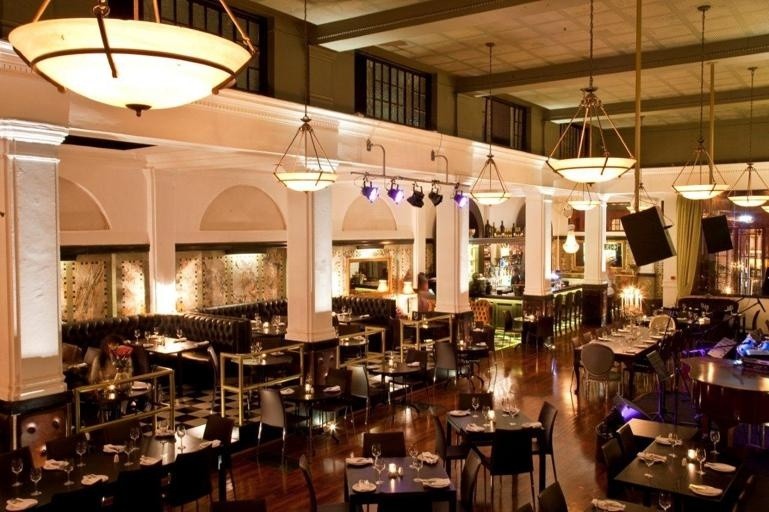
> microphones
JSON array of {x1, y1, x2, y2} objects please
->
[{"x1": 755, "y1": 296, "x2": 767, "y2": 313}]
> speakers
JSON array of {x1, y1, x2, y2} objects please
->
[
  {"x1": 620, "y1": 206, "x2": 678, "y2": 268},
  {"x1": 701, "y1": 214, "x2": 734, "y2": 253}
]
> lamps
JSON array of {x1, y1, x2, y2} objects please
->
[{"x1": 562, "y1": 218, "x2": 580, "y2": 255}]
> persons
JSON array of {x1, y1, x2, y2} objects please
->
[
  {"x1": 420, "y1": 279, "x2": 436, "y2": 295},
  {"x1": 510, "y1": 267, "x2": 520, "y2": 290}
]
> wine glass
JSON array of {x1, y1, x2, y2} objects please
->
[
  {"x1": 645, "y1": 431, "x2": 720, "y2": 512},
  {"x1": 11, "y1": 417, "x2": 186, "y2": 497},
  {"x1": 241, "y1": 313, "x2": 281, "y2": 330},
  {"x1": 372, "y1": 443, "x2": 423, "y2": 484},
  {"x1": 341, "y1": 305, "x2": 353, "y2": 321},
  {"x1": 602, "y1": 323, "x2": 659, "y2": 354},
  {"x1": 134, "y1": 327, "x2": 183, "y2": 346},
  {"x1": 472, "y1": 395, "x2": 520, "y2": 427}
]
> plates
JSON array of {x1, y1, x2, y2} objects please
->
[
  {"x1": 349, "y1": 454, "x2": 450, "y2": 493},
  {"x1": 451, "y1": 410, "x2": 530, "y2": 432}
]
